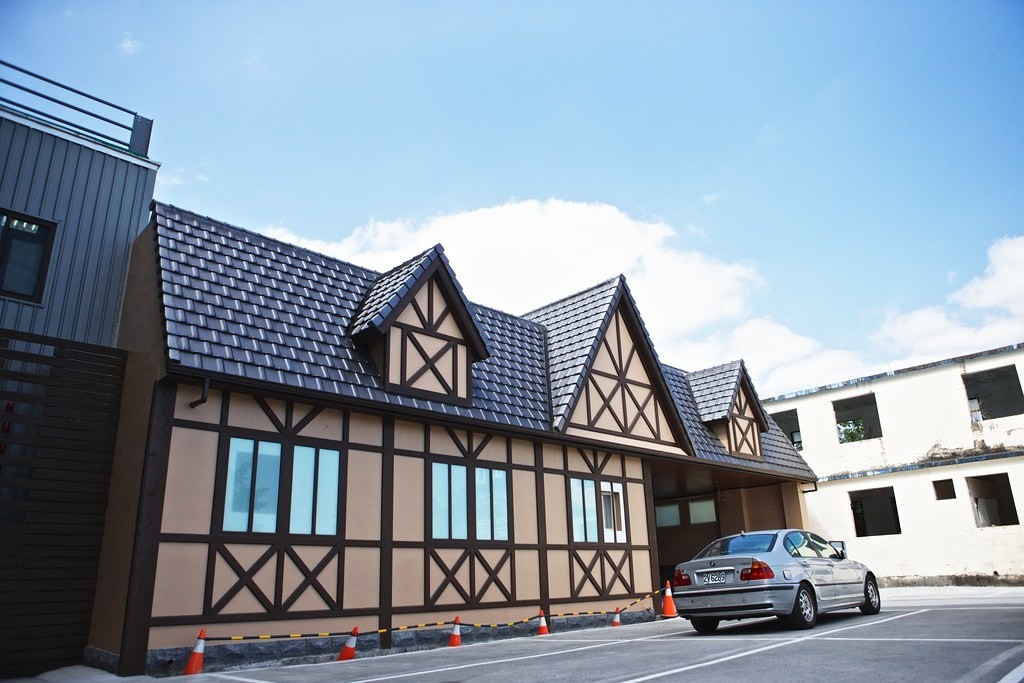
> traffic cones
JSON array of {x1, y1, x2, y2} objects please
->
[
  {"x1": 538, "y1": 610, "x2": 548, "y2": 635},
  {"x1": 183, "y1": 628, "x2": 206, "y2": 675},
  {"x1": 661, "y1": 580, "x2": 679, "y2": 617},
  {"x1": 336, "y1": 626, "x2": 359, "y2": 660},
  {"x1": 447, "y1": 616, "x2": 461, "y2": 646},
  {"x1": 611, "y1": 607, "x2": 621, "y2": 626}
]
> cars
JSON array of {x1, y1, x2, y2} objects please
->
[{"x1": 672, "y1": 528, "x2": 880, "y2": 633}]
